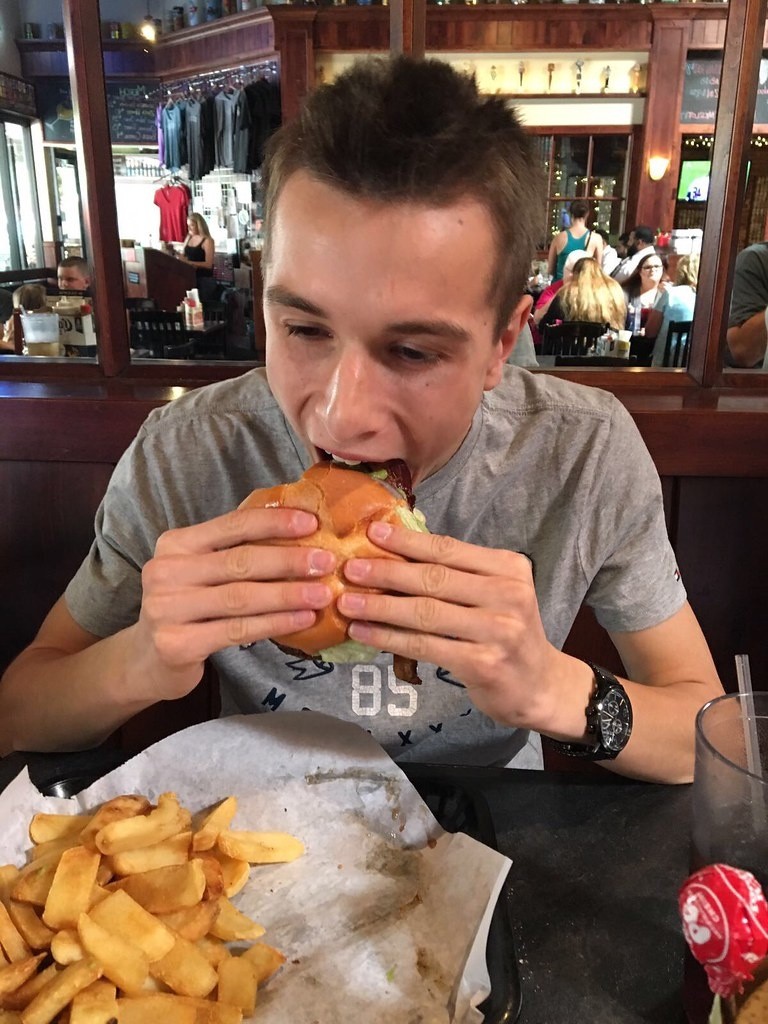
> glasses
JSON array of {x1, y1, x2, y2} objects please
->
[{"x1": 642, "y1": 264, "x2": 664, "y2": 273}]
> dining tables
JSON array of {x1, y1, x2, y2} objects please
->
[{"x1": 0, "y1": 748, "x2": 694, "y2": 1024}]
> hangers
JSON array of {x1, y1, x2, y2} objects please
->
[
  {"x1": 150, "y1": 170, "x2": 191, "y2": 190},
  {"x1": 157, "y1": 65, "x2": 264, "y2": 109}
]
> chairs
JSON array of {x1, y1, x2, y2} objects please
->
[
  {"x1": 123, "y1": 295, "x2": 231, "y2": 361},
  {"x1": 539, "y1": 319, "x2": 692, "y2": 368}
]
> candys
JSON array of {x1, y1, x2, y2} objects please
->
[{"x1": 679, "y1": 862, "x2": 768, "y2": 997}]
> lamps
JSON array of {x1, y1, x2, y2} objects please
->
[{"x1": 647, "y1": 156, "x2": 670, "y2": 180}]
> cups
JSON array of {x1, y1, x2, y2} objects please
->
[
  {"x1": 694, "y1": 686, "x2": 768, "y2": 899},
  {"x1": 20, "y1": 311, "x2": 60, "y2": 356}
]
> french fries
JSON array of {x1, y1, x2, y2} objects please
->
[{"x1": 0, "y1": 792, "x2": 306, "y2": 1024}]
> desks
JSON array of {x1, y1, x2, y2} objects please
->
[{"x1": 137, "y1": 320, "x2": 229, "y2": 361}]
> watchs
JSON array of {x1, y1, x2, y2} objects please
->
[{"x1": 552, "y1": 660, "x2": 636, "y2": 763}]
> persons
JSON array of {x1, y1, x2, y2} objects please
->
[
  {"x1": 0, "y1": 187, "x2": 768, "y2": 366},
  {"x1": 0, "y1": 56, "x2": 768, "y2": 786}
]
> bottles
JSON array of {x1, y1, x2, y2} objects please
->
[{"x1": 20, "y1": 0, "x2": 259, "y2": 42}]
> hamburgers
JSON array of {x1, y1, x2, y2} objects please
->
[{"x1": 236, "y1": 459, "x2": 432, "y2": 683}]
